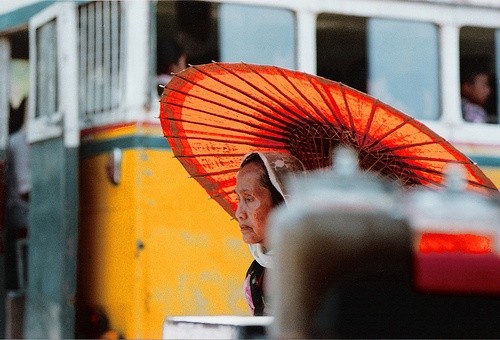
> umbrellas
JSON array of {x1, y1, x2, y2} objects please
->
[{"x1": 155, "y1": 60, "x2": 500, "y2": 253}]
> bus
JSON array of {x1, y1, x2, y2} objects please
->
[{"x1": 0, "y1": 0, "x2": 500, "y2": 340}]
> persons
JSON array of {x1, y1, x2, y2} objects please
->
[
  {"x1": 157, "y1": 48, "x2": 188, "y2": 97},
  {"x1": 462, "y1": 71, "x2": 491, "y2": 122},
  {"x1": 234, "y1": 151, "x2": 306, "y2": 316}
]
